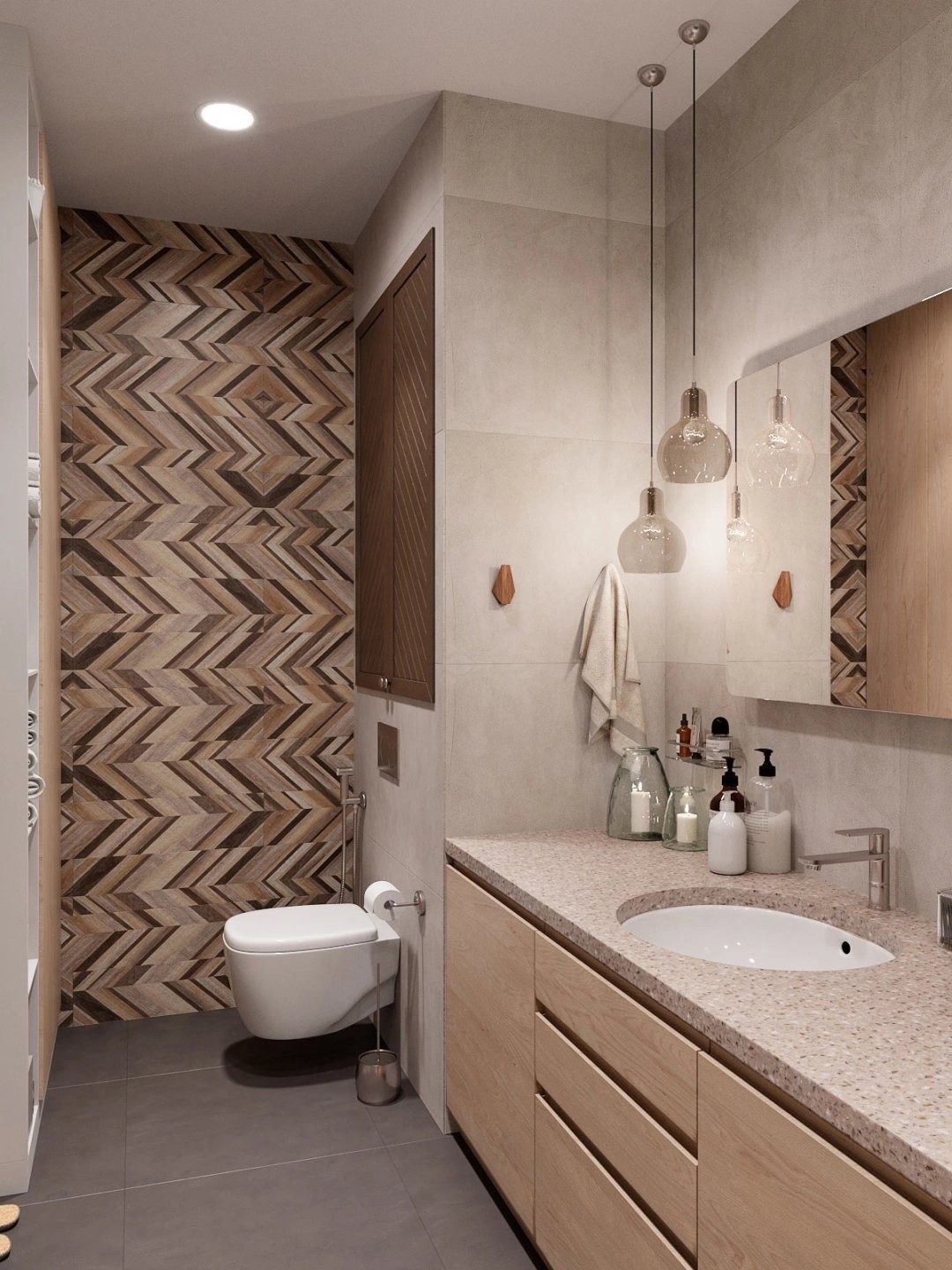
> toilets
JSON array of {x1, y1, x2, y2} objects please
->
[{"x1": 221, "y1": 901, "x2": 401, "y2": 1044}]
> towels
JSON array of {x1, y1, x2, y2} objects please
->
[{"x1": 575, "y1": 562, "x2": 651, "y2": 782}]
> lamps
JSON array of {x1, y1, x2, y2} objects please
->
[
  {"x1": 727, "y1": 380, "x2": 752, "y2": 546},
  {"x1": 659, "y1": 20, "x2": 734, "y2": 484},
  {"x1": 615, "y1": 65, "x2": 690, "y2": 575},
  {"x1": 749, "y1": 362, "x2": 813, "y2": 490}
]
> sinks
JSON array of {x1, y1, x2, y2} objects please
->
[{"x1": 613, "y1": 885, "x2": 904, "y2": 975}]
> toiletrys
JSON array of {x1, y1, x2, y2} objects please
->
[
  {"x1": 709, "y1": 755, "x2": 751, "y2": 822},
  {"x1": 704, "y1": 716, "x2": 735, "y2": 764},
  {"x1": 676, "y1": 712, "x2": 691, "y2": 757},
  {"x1": 747, "y1": 748, "x2": 794, "y2": 876},
  {"x1": 689, "y1": 706, "x2": 704, "y2": 760},
  {"x1": 707, "y1": 788, "x2": 748, "y2": 876}
]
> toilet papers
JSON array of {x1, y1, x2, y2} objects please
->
[{"x1": 363, "y1": 881, "x2": 404, "y2": 922}]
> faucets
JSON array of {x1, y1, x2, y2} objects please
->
[{"x1": 796, "y1": 826, "x2": 892, "y2": 913}]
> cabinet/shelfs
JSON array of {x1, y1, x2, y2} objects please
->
[
  {"x1": 442, "y1": 864, "x2": 952, "y2": 1270},
  {"x1": 355, "y1": 226, "x2": 435, "y2": 704}
]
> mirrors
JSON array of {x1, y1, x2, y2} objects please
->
[{"x1": 723, "y1": 270, "x2": 952, "y2": 755}]
